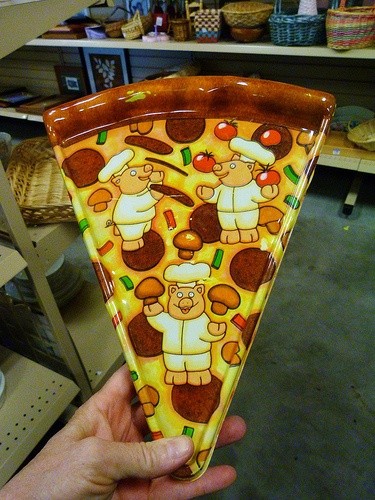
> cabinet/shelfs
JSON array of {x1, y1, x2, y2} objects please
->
[
  {"x1": 0, "y1": 1, "x2": 375, "y2": 213},
  {"x1": 0, "y1": 107, "x2": 127, "y2": 487}
]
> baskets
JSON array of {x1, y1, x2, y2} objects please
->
[
  {"x1": 5, "y1": 135, "x2": 79, "y2": 225},
  {"x1": 347, "y1": 115, "x2": 375, "y2": 151},
  {"x1": 101, "y1": 0, "x2": 375, "y2": 51}
]
